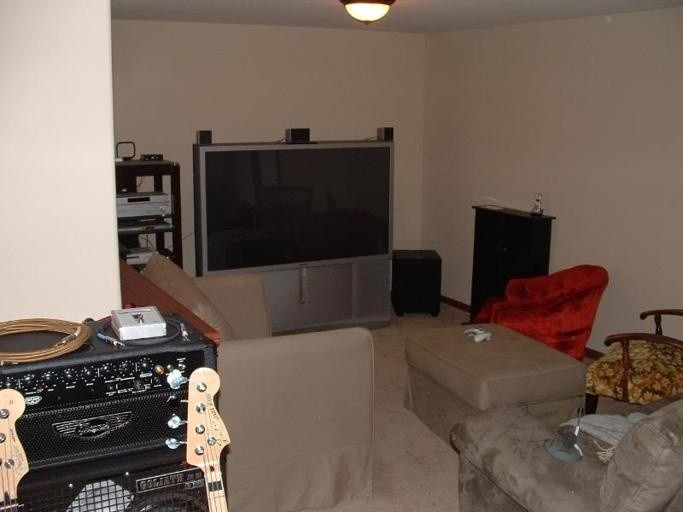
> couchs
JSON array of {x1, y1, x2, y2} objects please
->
[
  {"x1": 141, "y1": 253, "x2": 375, "y2": 511},
  {"x1": 472, "y1": 265, "x2": 609, "y2": 362},
  {"x1": 450, "y1": 391, "x2": 683, "y2": 512}
]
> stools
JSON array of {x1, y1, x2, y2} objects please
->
[{"x1": 405, "y1": 324, "x2": 585, "y2": 457}]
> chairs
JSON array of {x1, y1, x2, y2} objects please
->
[{"x1": 585, "y1": 309, "x2": 683, "y2": 416}]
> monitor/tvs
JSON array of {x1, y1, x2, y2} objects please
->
[{"x1": 193, "y1": 139, "x2": 397, "y2": 278}]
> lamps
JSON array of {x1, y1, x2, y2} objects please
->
[{"x1": 340, "y1": 0, "x2": 396, "y2": 26}]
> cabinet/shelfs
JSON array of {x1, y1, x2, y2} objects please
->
[
  {"x1": 115, "y1": 159, "x2": 183, "y2": 272},
  {"x1": 461, "y1": 206, "x2": 557, "y2": 324}
]
